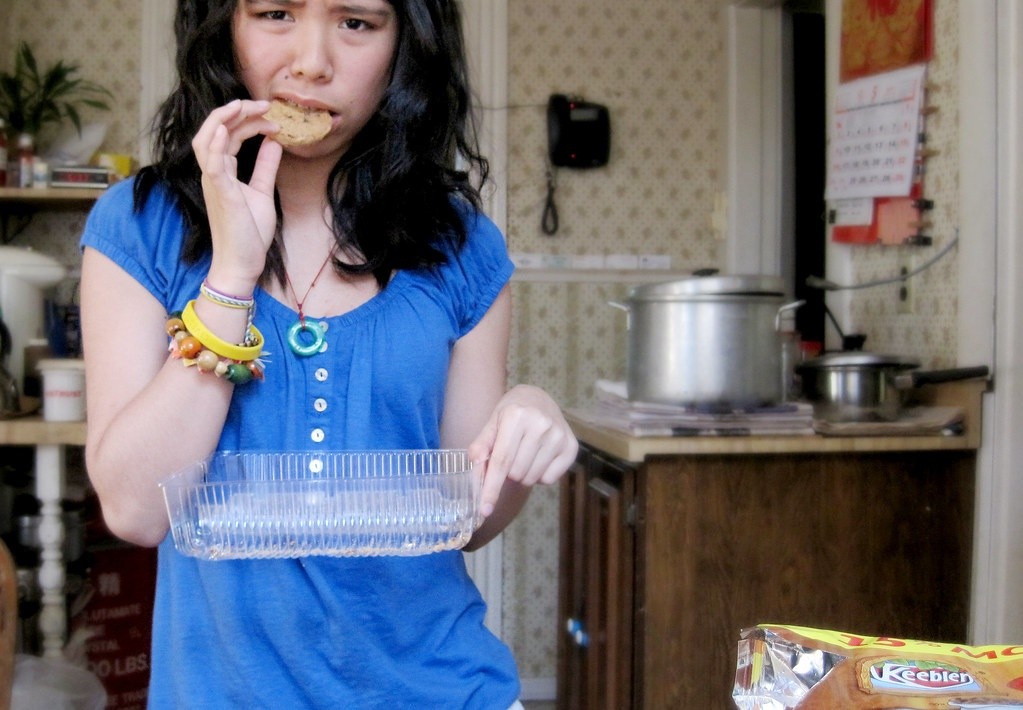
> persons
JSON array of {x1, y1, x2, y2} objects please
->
[{"x1": 79, "y1": 0, "x2": 579, "y2": 709}]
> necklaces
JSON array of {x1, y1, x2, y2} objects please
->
[{"x1": 273, "y1": 219, "x2": 346, "y2": 357}]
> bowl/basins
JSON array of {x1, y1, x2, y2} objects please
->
[{"x1": 158, "y1": 447, "x2": 481, "y2": 562}]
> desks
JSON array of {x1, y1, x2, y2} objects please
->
[{"x1": 1, "y1": 398, "x2": 88, "y2": 671}]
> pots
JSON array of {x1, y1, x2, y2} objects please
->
[
  {"x1": 607, "y1": 269, "x2": 806, "y2": 411},
  {"x1": 794, "y1": 333, "x2": 989, "y2": 437}
]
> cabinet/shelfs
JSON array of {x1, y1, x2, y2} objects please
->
[{"x1": 558, "y1": 410, "x2": 978, "y2": 709}]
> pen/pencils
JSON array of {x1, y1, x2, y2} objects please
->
[{"x1": 634, "y1": 424, "x2": 750, "y2": 440}]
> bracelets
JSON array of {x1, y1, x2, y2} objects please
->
[{"x1": 165, "y1": 277, "x2": 264, "y2": 386}]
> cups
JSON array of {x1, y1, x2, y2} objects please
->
[{"x1": 35, "y1": 358, "x2": 86, "y2": 423}]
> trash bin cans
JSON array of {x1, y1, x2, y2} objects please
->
[{"x1": 10, "y1": 653, "x2": 108, "y2": 710}]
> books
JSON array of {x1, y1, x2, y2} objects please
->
[{"x1": 561, "y1": 378, "x2": 965, "y2": 436}]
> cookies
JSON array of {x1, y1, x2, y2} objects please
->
[{"x1": 259, "y1": 99, "x2": 331, "y2": 145}]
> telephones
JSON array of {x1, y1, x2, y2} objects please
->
[{"x1": 550, "y1": 93, "x2": 611, "y2": 169}]
